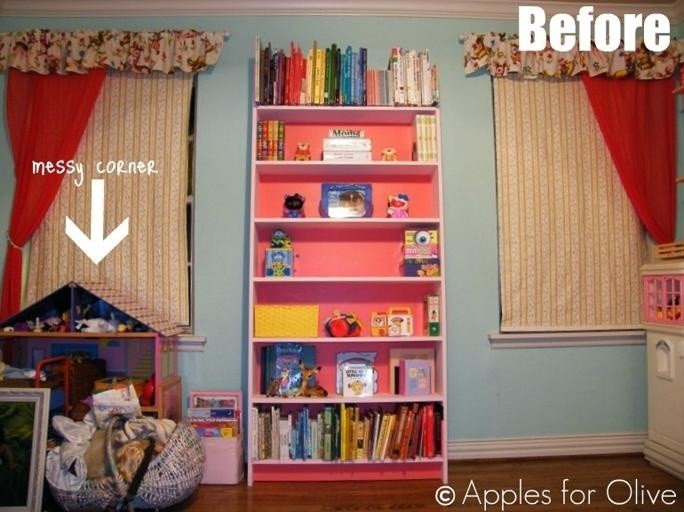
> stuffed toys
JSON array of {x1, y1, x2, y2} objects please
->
[
  {"x1": 271, "y1": 229, "x2": 288, "y2": 248},
  {"x1": 293, "y1": 360, "x2": 327, "y2": 398},
  {"x1": 386, "y1": 193, "x2": 411, "y2": 218},
  {"x1": 324, "y1": 309, "x2": 362, "y2": 337},
  {"x1": 283, "y1": 193, "x2": 307, "y2": 219}
]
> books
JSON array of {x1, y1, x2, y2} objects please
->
[
  {"x1": 253, "y1": 38, "x2": 440, "y2": 106},
  {"x1": 257, "y1": 119, "x2": 284, "y2": 161},
  {"x1": 399, "y1": 358, "x2": 430, "y2": 396},
  {"x1": 251, "y1": 403, "x2": 440, "y2": 461},
  {"x1": 412, "y1": 114, "x2": 438, "y2": 161}
]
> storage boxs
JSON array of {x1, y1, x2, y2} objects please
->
[{"x1": 252, "y1": 302, "x2": 320, "y2": 339}]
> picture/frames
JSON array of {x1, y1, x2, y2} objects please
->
[
  {"x1": 188, "y1": 391, "x2": 242, "y2": 411},
  {"x1": 320, "y1": 182, "x2": 372, "y2": 218},
  {"x1": 1, "y1": 386, "x2": 51, "y2": 512}
]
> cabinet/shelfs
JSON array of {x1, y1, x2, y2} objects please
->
[
  {"x1": 246, "y1": 101, "x2": 450, "y2": 488},
  {"x1": 639, "y1": 260, "x2": 683, "y2": 479},
  {"x1": 1, "y1": 323, "x2": 183, "y2": 427}
]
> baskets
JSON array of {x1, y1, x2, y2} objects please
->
[{"x1": 45, "y1": 415, "x2": 206, "y2": 512}]
